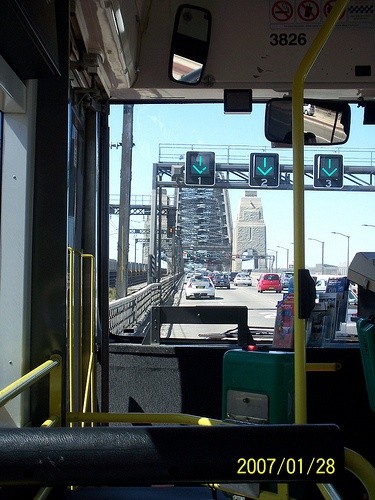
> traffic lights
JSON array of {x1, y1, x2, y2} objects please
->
[
  {"x1": 185, "y1": 151, "x2": 216, "y2": 187},
  {"x1": 249, "y1": 152, "x2": 280, "y2": 189},
  {"x1": 313, "y1": 153, "x2": 344, "y2": 190}
]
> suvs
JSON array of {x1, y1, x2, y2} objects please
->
[{"x1": 279, "y1": 271, "x2": 294, "y2": 291}]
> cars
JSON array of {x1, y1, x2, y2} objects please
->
[
  {"x1": 256, "y1": 273, "x2": 282, "y2": 293},
  {"x1": 183, "y1": 275, "x2": 215, "y2": 300},
  {"x1": 183, "y1": 267, "x2": 238, "y2": 284},
  {"x1": 213, "y1": 274, "x2": 230, "y2": 289},
  {"x1": 233, "y1": 272, "x2": 252, "y2": 286},
  {"x1": 314, "y1": 279, "x2": 358, "y2": 336},
  {"x1": 302, "y1": 103, "x2": 315, "y2": 116}
]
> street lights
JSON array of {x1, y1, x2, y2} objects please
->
[
  {"x1": 267, "y1": 248, "x2": 278, "y2": 273},
  {"x1": 276, "y1": 245, "x2": 288, "y2": 272},
  {"x1": 330, "y1": 231, "x2": 350, "y2": 268},
  {"x1": 307, "y1": 238, "x2": 324, "y2": 275}
]
